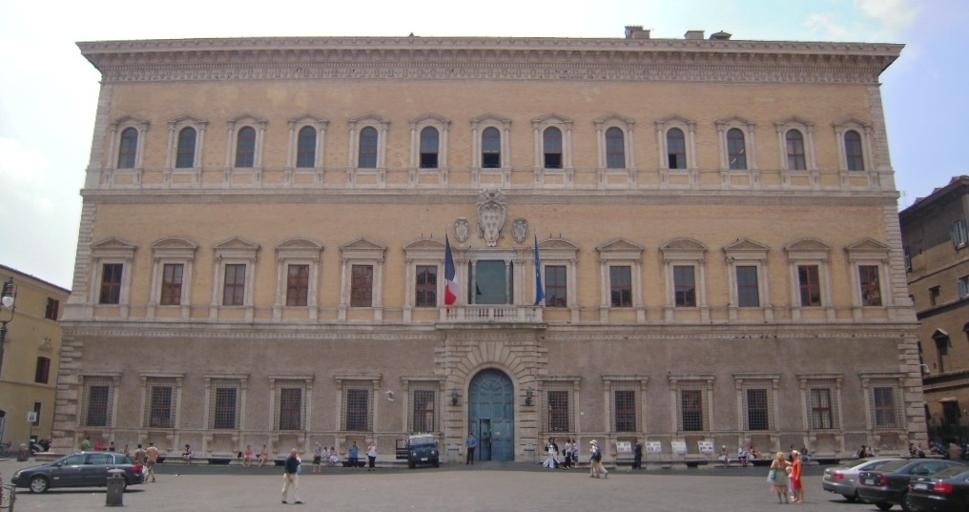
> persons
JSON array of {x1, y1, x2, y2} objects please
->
[
  {"x1": 81, "y1": 435, "x2": 377, "y2": 503},
  {"x1": 720, "y1": 444, "x2": 807, "y2": 503},
  {"x1": 859, "y1": 440, "x2": 963, "y2": 460},
  {"x1": 543, "y1": 437, "x2": 642, "y2": 479},
  {"x1": 465, "y1": 432, "x2": 477, "y2": 464}
]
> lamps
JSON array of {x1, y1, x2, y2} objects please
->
[
  {"x1": 526, "y1": 387, "x2": 533, "y2": 405},
  {"x1": 451, "y1": 388, "x2": 459, "y2": 406}
]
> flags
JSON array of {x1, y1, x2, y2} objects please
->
[
  {"x1": 533, "y1": 235, "x2": 545, "y2": 306},
  {"x1": 445, "y1": 235, "x2": 458, "y2": 304}
]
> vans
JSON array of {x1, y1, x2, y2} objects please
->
[{"x1": 395, "y1": 433, "x2": 441, "y2": 470}]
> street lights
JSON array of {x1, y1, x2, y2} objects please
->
[{"x1": 0, "y1": 274, "x2": 21, "y2": 372}]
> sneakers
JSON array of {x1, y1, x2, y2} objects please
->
[
  {"x1": 542, "y1": 463, "x2": 577, "y2": 471},
  {"x1": 777, "y1": 495, "x2": 803, "y2": 505},
  {"x1": 589, "y1": 470, "x2": 609, "y2": 480},
  {"x1": 281, "y1": 499, "x2": 306, "y2": 506}
]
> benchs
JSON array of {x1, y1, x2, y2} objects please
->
[
  {"x1": 143, "y1": 455, "x2": 369, "y2": 467},
  {"x1": 615, "y1": 457, "x2": 841, "y2": 470}
]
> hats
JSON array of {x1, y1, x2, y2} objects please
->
[{"x1": 589, "y1": 440, "x2": 598, "y2": 446}]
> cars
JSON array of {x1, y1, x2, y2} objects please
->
[
  {"x1": 853, "y1": 458, "x2": 965, "y2": 510},
  {"x1": 819, "y1": 456, "x2": 904, "y2": 504},
  {"x1": 903, "y1": 460, "x2": 969, "y2": 512},
  {"x1": 8, "y1": 450, "x2": 147, "y2": 495}
]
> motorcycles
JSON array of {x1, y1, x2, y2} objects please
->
[{"x1": 29, "y1": 435, "x2": 49, "y2": 456}]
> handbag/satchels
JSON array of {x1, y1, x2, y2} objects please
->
[{"x1": 769, "y1": 468, "x2": 777, "y2": 481}]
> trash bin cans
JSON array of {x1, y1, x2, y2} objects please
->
[
  {"x1": 105, "y1": 468, "x2": 125, "y2": 506},
  {"x1": 17, "y1": 443, "x2": 29, "y2": 461}
]
